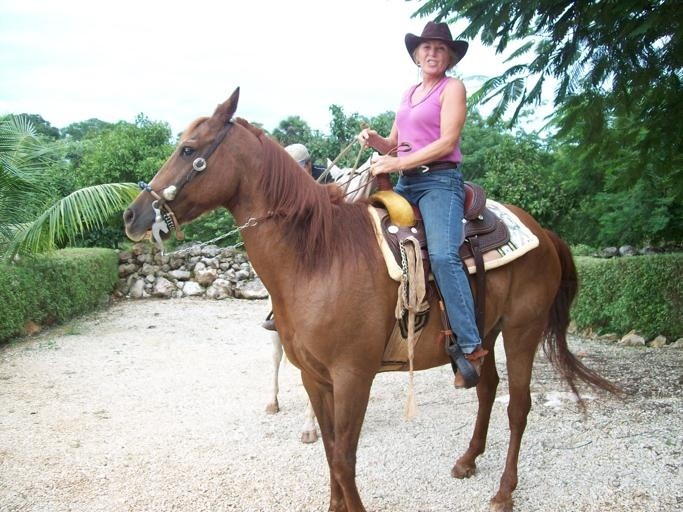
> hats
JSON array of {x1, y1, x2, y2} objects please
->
[
  {"x1": 404, "y1": 21, "x2": 468, "y2": 70},
  {"x1": 284, "y1": 144, "x2": 309, "y2": 167}
]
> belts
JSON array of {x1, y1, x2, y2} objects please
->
[{"x1": 403, "y1": 161, "x2": 456, "y2": 176}]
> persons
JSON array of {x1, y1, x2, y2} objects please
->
[
  {"x1": 262, "y1": 143, "x2": 332, "y2": 330},
  {"x1": 358, "y1": 22, "x2": 485, "y2": 389}
]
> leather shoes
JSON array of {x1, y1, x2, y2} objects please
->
[
  {"x1": 262, "y1": 318, "x2": 275, "y2": 332},
  {"x1": 454, "y1": 348, "x2": 485, "y2": 390}
]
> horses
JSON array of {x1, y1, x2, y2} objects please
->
[
  {"x1": 123, "y1": 88, "x2": 639, "y2": 511},
  {"x1": 265, "y1": 151, "x2": 383, "y2": 444}
]
